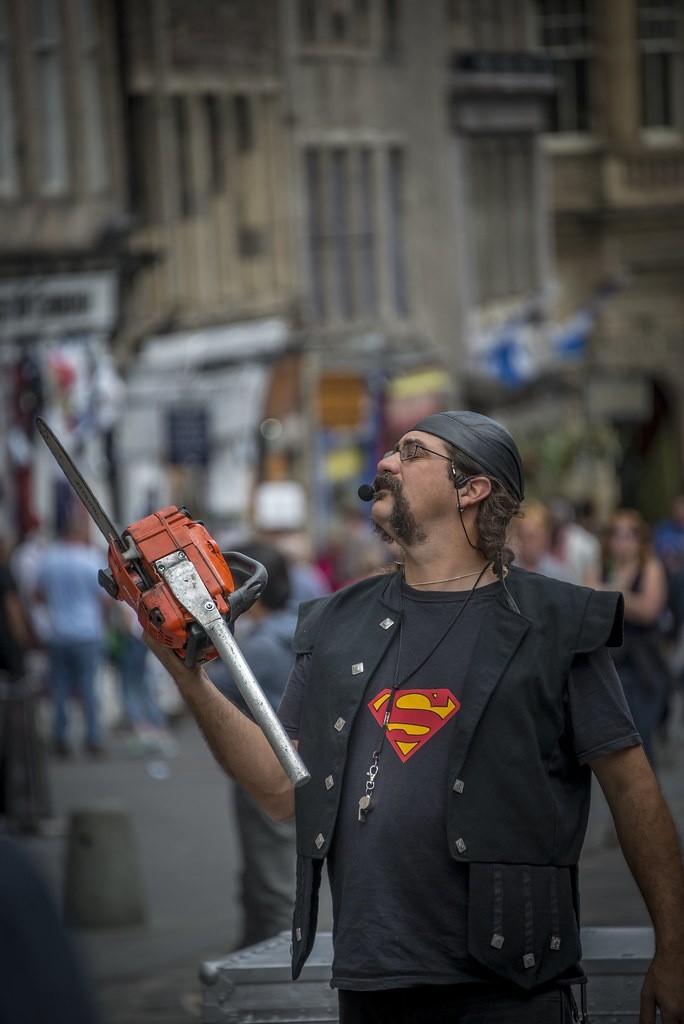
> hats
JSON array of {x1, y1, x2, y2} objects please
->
[{"x1": 405, "y1": 410, "x2": 525, "y2": 502}]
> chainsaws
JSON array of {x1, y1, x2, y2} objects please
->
[{"x1": 34, "y1": 416, "x2": 314, "y2": 789}]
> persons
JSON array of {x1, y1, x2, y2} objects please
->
[
  {"x1": 207, "y1": 544, "x2": 303, "y2": 955},
  {"x1": 142, "y1": 411, "x2": 684, "y2": 1024},
  {"x1": 0, "y1": 482, "x2": 402, "y2": 831},
  {"x1": 502, "y1": 497, "x2": 684, "y2": 764}
]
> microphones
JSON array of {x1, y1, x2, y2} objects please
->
[{"x1": 356, "y1": 483, "x2": 376, "y2": 503}]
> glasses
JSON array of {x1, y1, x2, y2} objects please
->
[{"x1": 382, "y1": 443, "x2": 453, "y2": 462}]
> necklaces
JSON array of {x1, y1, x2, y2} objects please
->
[{"x1": 404, "y1": 564, "x2": 501, "y2": 586}]
